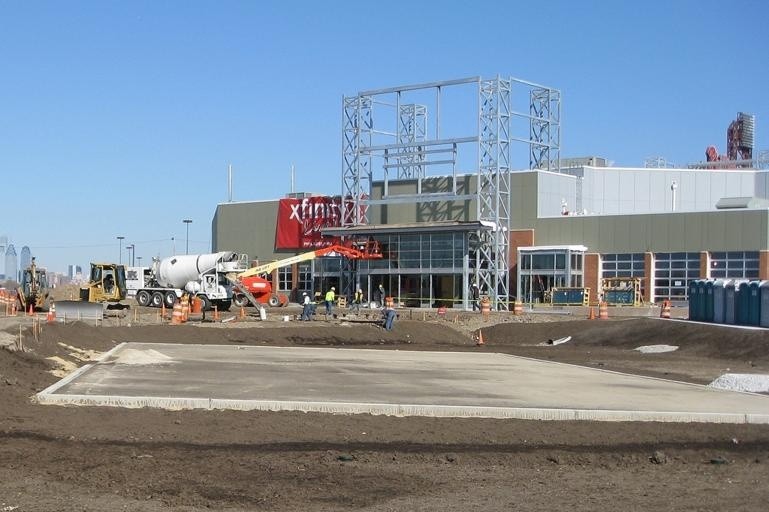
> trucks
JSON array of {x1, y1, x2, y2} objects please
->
[{"x1": 127, "y1": 248, "x2": 245, "y2": 311}]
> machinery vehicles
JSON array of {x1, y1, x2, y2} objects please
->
[
  {"x1": 233, "y1": 243, "x2": 382, "y2": 307},
  {"x1": 53, "y1": 262, "x2": 130, "y2": 322},
  {"x1": 16, "y1": 256, "x2": 49, "y2": 311}
]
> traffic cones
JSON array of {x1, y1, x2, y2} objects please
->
[
  {"x1": 46, "y1": 296, "x2": 56, "y2": 322},
  {"x1": 589, "y1": 308, "x2": 595, "y2": 319},
  {"x1": 240, "y1": 306, "x2": 245, "y2": 320},
  {"x1": 29, "y1": 304, "x2": 33, "y2": 315},
  {"x1": 212, "y1": 305, "x2": 219, "y2": 318},
  {"x1": 477, "y1": 329, "x2": 485, "y2": 345},
  {"x1": 1, "y1": 288, "x2": 19, "y2": 316},
  {"x1": 160, "y1": 294, "x2": 201, "y2": 324}
]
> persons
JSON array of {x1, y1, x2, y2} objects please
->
[
  {"x1": 350, "y1": 288, "x2": 366, "y2": 310},
  {"x1": 301, "y1": 292, "x2": 313, "y2": 321},
  {"x1": 381, "y1": 308, "x2": 396, "y2": 331},
  {"x1": 377, "y1": 284, "x2": 385, "y2": 308},
  {"x1": 180, "y1": 293, "x2": 189, "y2": 322},
  {"x1": 324, "y1": 287, "x2": 335, "y2": 315},
  {"x1": 472, "y1": 284, "x2": 481, "y2": 311}
]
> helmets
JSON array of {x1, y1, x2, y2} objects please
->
[
  {"x1": 331, "y1": 287, "x2": 335, "y2": 290},
  {"x1": 359, "y1": 289, "x2": 362, "y2": 293},
  {"x1": 473, "y1": 284, "x2": 476, "y2": 286},
  {"x1": 303, "y1": 293, "x2": 307, "y2": 295},
  {"x1": 379, "y1": 285, "x2": 382, "y2": 287}
]
